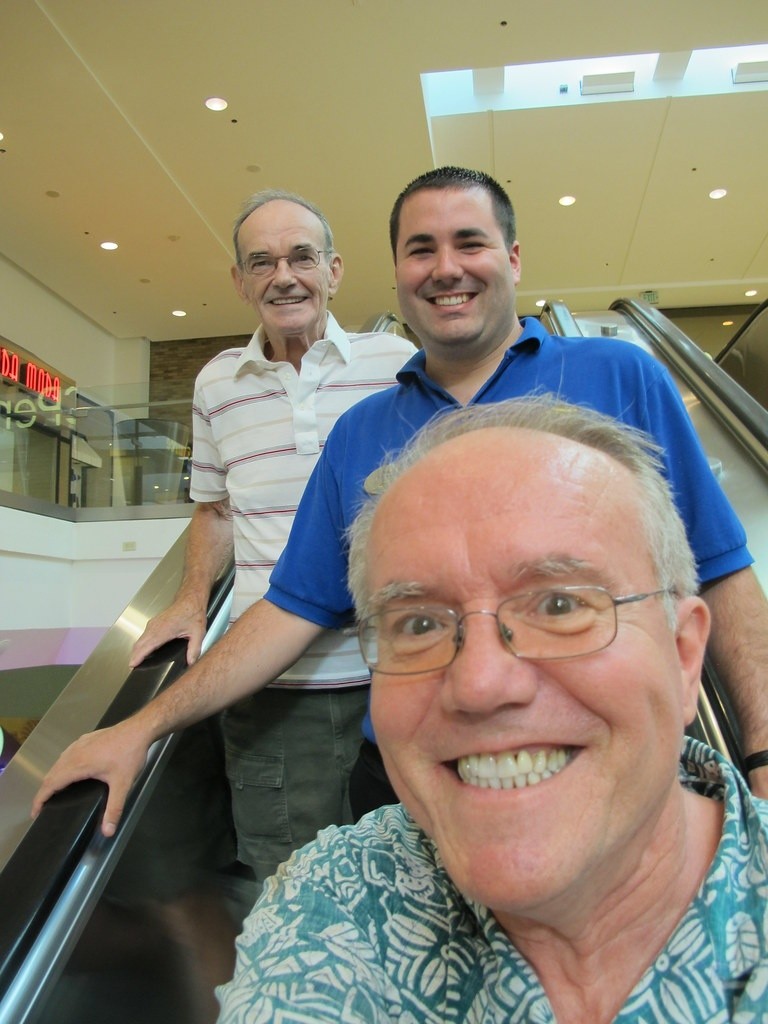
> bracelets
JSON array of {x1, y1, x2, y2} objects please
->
[{"x1": 745, "y1": 750, "x2": 767, "y2": 775}]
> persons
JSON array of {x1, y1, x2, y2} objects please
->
[
  {"x1": 28, "y1": 168, "x2": 768, "y2": 841},
  {"x1": 130, "y1": 190, "x2": 418, "y2": 883},
  {"x1": 218, "y1": 392, "x2": 768, "y2": 1024}
]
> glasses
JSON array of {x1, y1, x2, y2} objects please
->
[
  {"x1": 358, "y1": 583, "x2": 681, "y2": 674},
  {"x1": 239, "y1": 248, "x2": 335, "y2": 274}
]
